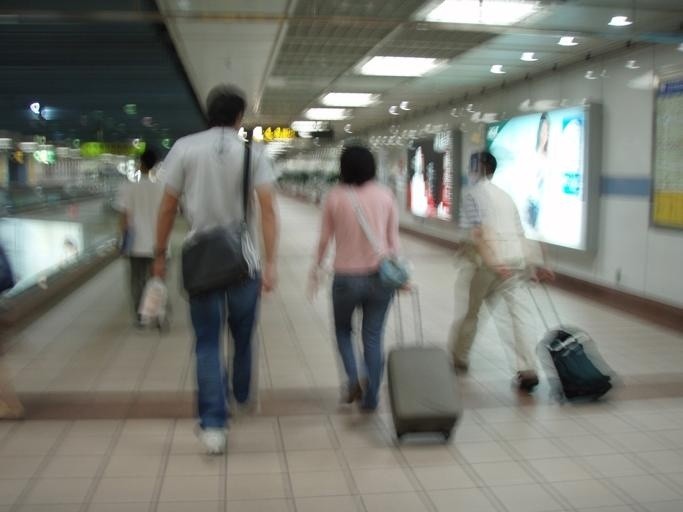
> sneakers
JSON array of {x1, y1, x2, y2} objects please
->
[{"x1": 195, "y1": 419, "x2": 227, "y2": 456}]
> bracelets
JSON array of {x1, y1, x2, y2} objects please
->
[{"x1": 151, "y1": 245, "x2": 168, "y2": 258}]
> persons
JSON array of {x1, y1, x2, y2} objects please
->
[
  {"x1": 447, "y1": 147, "x2": 536, "y2": 393},
  {"x1": 108, "y1": 144, "x2": 172, "y2": 329},
  {"x1": 151, "y1": 80, "x2": 279, "y2": 453},
  {"x1": 304, "y1": 143, "x2": 409, "y2": 414},
  {"x1": 517, "y1": 114, "x2": 562, "y2": 221},
  {"x1": 0, "y1": 242, "x2": 26, "y2": 422}
]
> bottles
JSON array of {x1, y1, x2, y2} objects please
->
[{"x1": 137, "y1": 266, "x2": 166, "y2": 328}]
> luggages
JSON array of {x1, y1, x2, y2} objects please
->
[
  {"x1": 388, "y1": 285, "x2": 462, "y2": 443},
  {"x1": 527, "y1": 274, "x2": 613, "y2": 400}
]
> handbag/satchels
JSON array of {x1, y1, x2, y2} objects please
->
[
  {"x1": 378, "y1": 258, "x2": 410, "y2": 291},
  {"x1": 181, "y1": 224, "x2": 251, "y2": 298}
]
